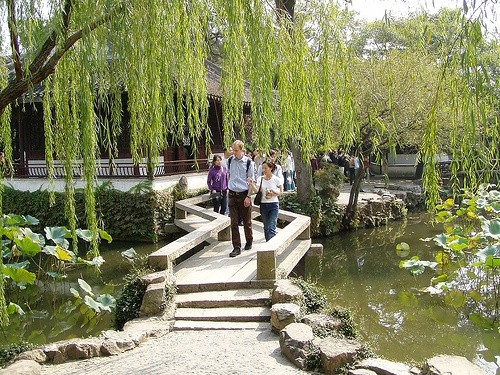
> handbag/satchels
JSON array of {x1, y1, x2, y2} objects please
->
[
  {"x1": 209, "y1": 192, "x2": 223, "y2": 204},
  {"x1": 254, "y1": 191, "x2": 262, "y2": 205}
]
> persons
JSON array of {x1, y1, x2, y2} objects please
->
[
  {"x1": 247, "y1": 162, "x2": 284, "y2": 243},
  {"x1": 246, "y1": 146, "x2": 376, "y2": 192},
  {"x1": 222, "y1": 146, "x2": 234, "y2": 215},
  {"x1": 207, "y1": 155, "x2": 229, "y2": 215},
  {"x1": 226, "y1": 139, "x2": 256, "y2": 257}
]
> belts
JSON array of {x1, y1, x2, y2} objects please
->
[{"x1": 228, "y1": 190, "x2": 248, "y2": 195}]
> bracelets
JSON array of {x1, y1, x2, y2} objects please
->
[{"x1": 247, "y1": 196, "x2": 251, "y2": 198}]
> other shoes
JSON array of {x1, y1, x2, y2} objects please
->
[
  {"x1": 229, "y1": 248, "x2": 241, "y2": 257},
  {"x1": 238, "y1": 223, "x2": 243, "y2": 226},
  {"x1": 245, "y1": 240, "x2": 252, "y2": 249}
]
